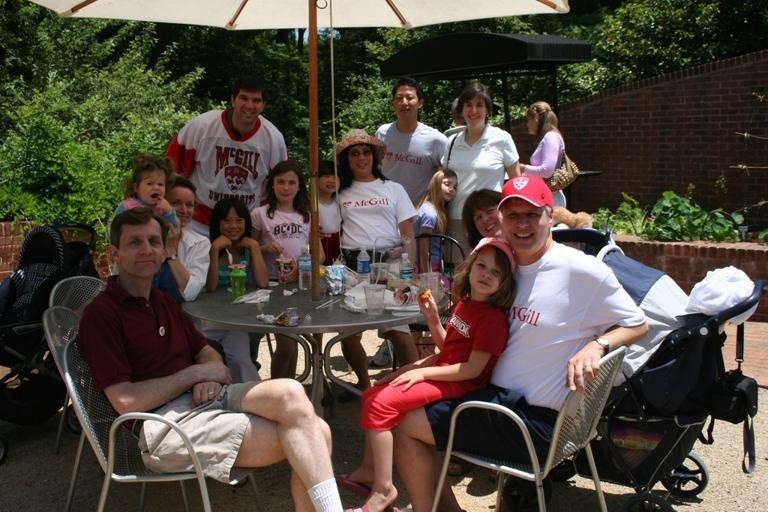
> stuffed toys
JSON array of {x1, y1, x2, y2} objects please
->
[{"x1": 549, "y1": 206, "x2": 594, "y2": 230}]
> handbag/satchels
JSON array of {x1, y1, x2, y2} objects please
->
[
  {"x1": 269, "y1": 246, "x2": 298, "y2": 286},
  {"x1": 710, "y1": 321, "x2": 763, "y2": 475},
  {"x1": 544, "y1": 152, "x2": 581, "y2": 193}
]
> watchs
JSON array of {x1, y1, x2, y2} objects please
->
[{"x1": 591, "y1": 337, "x2": 611, "y2": 356}]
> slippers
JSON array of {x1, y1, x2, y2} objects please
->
[
  {"x1": 334, "y1": 472, "x2": 371, "y2": 496},
  {"x1": 342, "y1": 502, "x2": 401, "y2": 512}
]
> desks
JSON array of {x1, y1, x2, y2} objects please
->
[{"x1": 179, "y1": 282, "x2": 457, "y2": 510}]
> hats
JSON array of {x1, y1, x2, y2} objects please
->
[
  {"x1": 335, "y1": 128, "x2": 387, "y2": 163},
  {"x1": 471, "y1": 236, "x2": 517, "y2": 269},
  {"x1": 496, "y1": 174, "x2": 556, "y2": 212}
]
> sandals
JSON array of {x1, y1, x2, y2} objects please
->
[{"x1": 354, "y1": 380, "x2": 370, "y2": 391}]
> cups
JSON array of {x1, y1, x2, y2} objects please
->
[
  {"x1": 419, "y1": 271, "x2": 445, "y2": 305},
  {"x1": 274, "y1": 257, "x2": 294, "y2": 284},
  {"x1": 361, "y1": 281, "x2": 387, "y2": 315},
  {"x1": 229, "y1": 266, "x2": 248, "y2": 300},
  {"x1": 227, "y1": 263, "x2": 246, "y2": 272}
]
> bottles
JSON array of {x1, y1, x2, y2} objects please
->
[
  {"x1": 370, "y1": 249, "x2": 415, "y2": 290},
  {"x1": 297, "y1": 246, "x2": 313, "y2": 292},
  {"x1": 355, "y1": 246, "x2": 372, "y2": 284},
  {"x1": 285, "y1": 306, "x2": 299, "y2": 328}
]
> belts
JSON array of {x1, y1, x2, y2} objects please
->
[{"x1": 132, "y1": 406, "x2": 159, "y2": 437}]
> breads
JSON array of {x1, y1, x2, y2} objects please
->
[{"x1": 417, "y1": 288, "x2": 431, "y2": 304}]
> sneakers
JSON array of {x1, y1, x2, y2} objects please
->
[{"x1": 369, "y1": 341, "x2": 391, "y2": 366}]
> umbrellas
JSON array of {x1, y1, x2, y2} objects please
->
[{"x1": 2, "y1": 0, "x2": 569, "y2": 356}]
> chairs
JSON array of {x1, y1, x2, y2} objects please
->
[
  {"x1": 43, "y1": 273, "x2": 265, "y2": 512},
  {"x1": 252, "y1": 233, "x2": 468, "y2": 376},
  {"x1": 430, "y1": 345, "x2": 628, "y2": 512}
]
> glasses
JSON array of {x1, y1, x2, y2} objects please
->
[{"x1": 351, "y1": 149, "x2": 371, "y2": 157}]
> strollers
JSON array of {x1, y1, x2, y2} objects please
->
[
  {"x1": 1, "y1": 215, "x2": 102, "y2": 460},
  {"x1": 500, "y1": 240, "x2": 765, "y2": 511}
]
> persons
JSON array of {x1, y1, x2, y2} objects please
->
[
  {"x1": 335, "y1": 237, "x2": 517, "y2": 512},
  {"x1": 75, "y1": 205, "x2": 345, "y2": 511},
  {"x1": 99, "y1": 73, "x2": 570, "y2": 408},
  {"x1": 393, "y1": 172, "x2": 649, "y2": 512}
]
